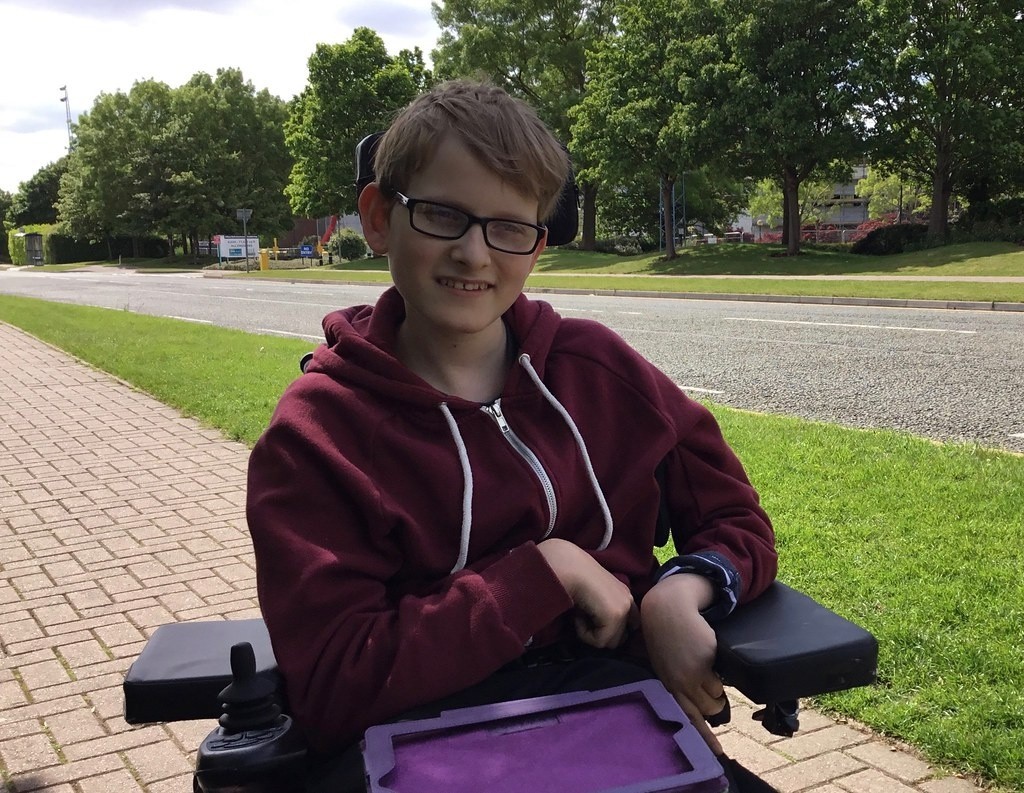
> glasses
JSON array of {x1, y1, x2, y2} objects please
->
[{"x1": 379, "y1": 182, "x2": 547, "y2": 256}]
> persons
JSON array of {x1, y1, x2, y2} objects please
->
[{"x1": 245, "y1": 81, "x2": 776, "y2": 793}]
